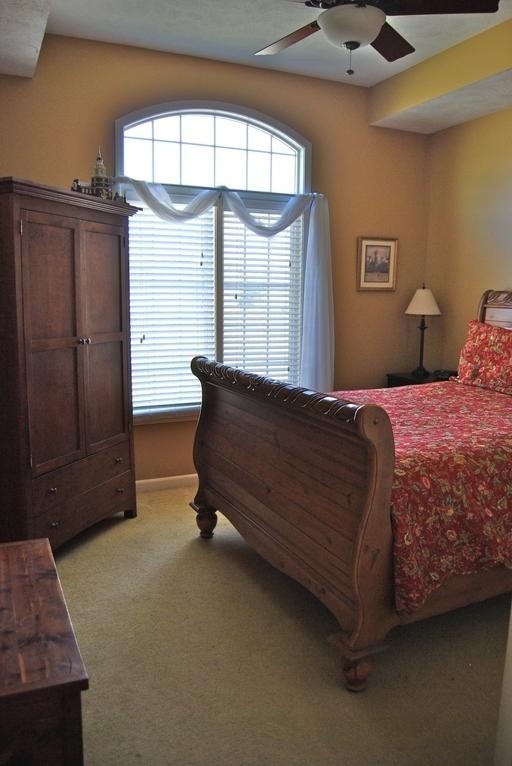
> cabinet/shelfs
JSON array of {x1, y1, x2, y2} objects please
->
[{"x1": 0, "y1": 173, "x2": 138, "y2": 544}]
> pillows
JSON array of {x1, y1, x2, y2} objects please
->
[{"x1": 448, "y1": 319, "x2": 512, "y2": 396}]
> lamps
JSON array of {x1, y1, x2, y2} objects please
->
[
  {"x1": 403, "y1": 288, "x2": 443, "y2": 379},
  {"x1": 316, "y1": 3, "x2": 387, "y2": 51}
]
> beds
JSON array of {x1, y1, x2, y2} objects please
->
[{"x1": 186, "y1": 290, "x2": 512, "y2": 696}]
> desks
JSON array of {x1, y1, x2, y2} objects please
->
[{"x1": 2, "y1": 539, "x2": 90, "y2": 763}]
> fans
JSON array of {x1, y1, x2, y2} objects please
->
[{"x1": 255, "y1": 0, "x2": 499, "y2": 79}]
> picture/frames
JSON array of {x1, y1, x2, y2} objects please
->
[{"x1": 355, "y1": 235, "x2": 399, "y2": 292}]
